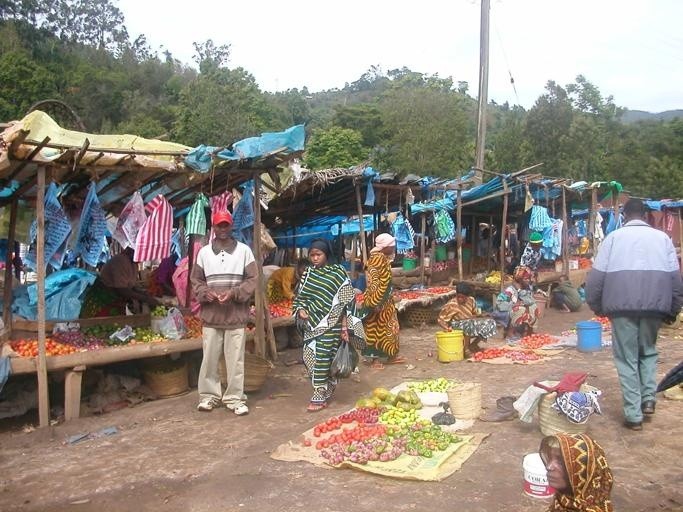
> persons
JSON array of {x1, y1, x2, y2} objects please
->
[
  {"x1": 520, "y1": 232, "x2": 543, "y2": 270},
  {"x1": 291, "y1": 238, "x2": 356, "y2": 412},
  {"x1": 585, "y1": 199, "x2": 683, "y2": 430},
  {"x1": 491, "y1": 266, "x2": 540, "y2": 335},
  {"x1": 362, "y1": 232, "x2": 406, "y2": 371},
  {"x1": 538, "y1": 430, "x2": 615, "y2": 512},
  {"x1": 552, "y1": 275, "x2": 581, "y2": 313},
  {"x1": 190, "y1": 209, "x2": 259, "y2": 416},
  {"x1": 266, "y1": 265, "x2": 307, "y2": 303},
  {"x1": 437, "y1": 282, "x2": 498, "y2": 354}
]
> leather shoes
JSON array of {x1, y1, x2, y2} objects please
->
[
  {"x1": 642, "y1": 401, "x2": 654, "y2": 416},
  {"x1": 623, "y1": 419, "x2": 642, "y2": 430}
]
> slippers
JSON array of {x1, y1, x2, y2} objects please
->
[{"x1": 306, "y1": 399, "x2": 328, "y2": 412}]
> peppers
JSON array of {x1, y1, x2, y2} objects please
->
[{"x1": 388, "y1": 420, "x2": 461, "y2": 458}]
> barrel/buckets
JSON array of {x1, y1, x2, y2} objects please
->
[
  {"x1": 575, "y1": 320, "x2": 602, "y2": 354},
  {"x1": 521, "y1": 451, "x2": 557, "y2": 500},
  {"x1": 434, "y1": 328, "x2": 466, "y2": 364}
]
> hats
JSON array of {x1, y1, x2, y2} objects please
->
[
  {"x1": 456, "y1": 281, "x2": 476, "y2": 297},
  {"x1": 512, "y1": 266, "x2": 533, "y2": 282},
  {"x1": 212, "y1": 208, "x2": 233, "y2": 226},
  {"x1": 369, "y1": 232, "x2": 397, "y2": 254}
]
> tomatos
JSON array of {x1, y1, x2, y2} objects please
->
[
  {"x1": 304, "y1": 440, "x2": 311, "y2": 446},
  {"x1": 314, "y1": 416, "x2": 342, "y2": 436},
  {"x1": 473, "y1": 346, "x2": 505, "y2": 360},
  {"x1": 317, "y1": 425, "x2": 385, "y2": 449},
  {"x1": 523, "y1": 332, "x2": 559, "y2": 347}
]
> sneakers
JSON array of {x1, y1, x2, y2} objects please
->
[
  {"x1": 226, "y1": 402, "x2": 250, "y2": 416},
  {"x1": 196, "y1": 394, "x2": 223, "y2": 412}
]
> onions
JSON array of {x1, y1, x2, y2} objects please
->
[
  {"x1": 506, "y1": 349, "x2": 542, "y2": 363},
  {"x1": 316, "y1": 438, "x2": 400, "y2": 464}
]
